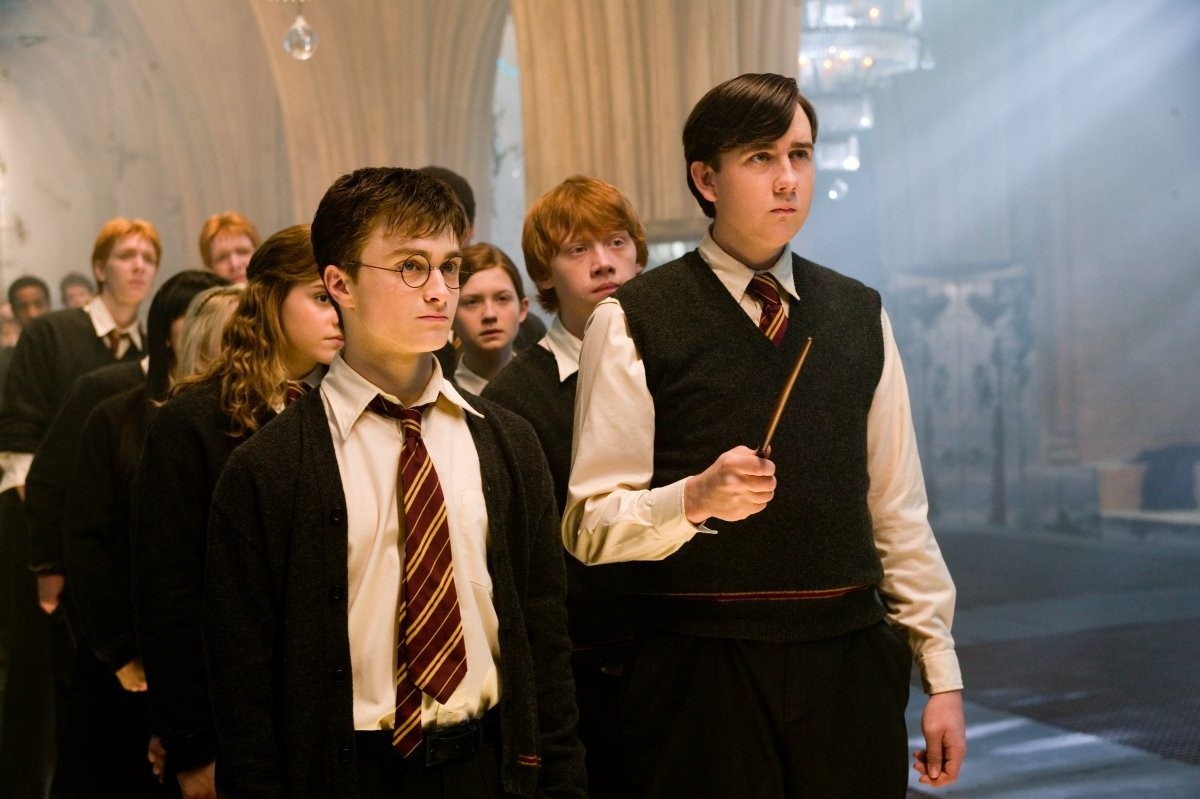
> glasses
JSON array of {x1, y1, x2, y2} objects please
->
[{"x1": 349, "y1": 251, "x2": 475, "y2": 290}]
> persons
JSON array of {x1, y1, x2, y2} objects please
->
[
  {"x1": 416, "y1": 170, "x2": 550, "y2": 404},
  {"x1": 562, "y1": 74, "x2": 966, "y2": 798},
  {"x1": 479, "y1": 176, "x2": 649, "y2": 522},
  {"x1": 202, "y1": 167, "x2": 590, "y2": 799},
  {"x1": 0, "y1": 211, "x2": 346, "y2": 799}
]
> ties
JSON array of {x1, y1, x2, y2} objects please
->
[
  {"x1": 369, "y1": 390, "x2": 468, "y2": 767},
  {"x1": 279, "y1": 380, "x2": 312, "y2": 410},
  {"x1": 747, "y1": 269, "x2": 790, "y2": 348},
  {"x1": 107, "y1": 328, "x2": 130, "y2": 354}
]
